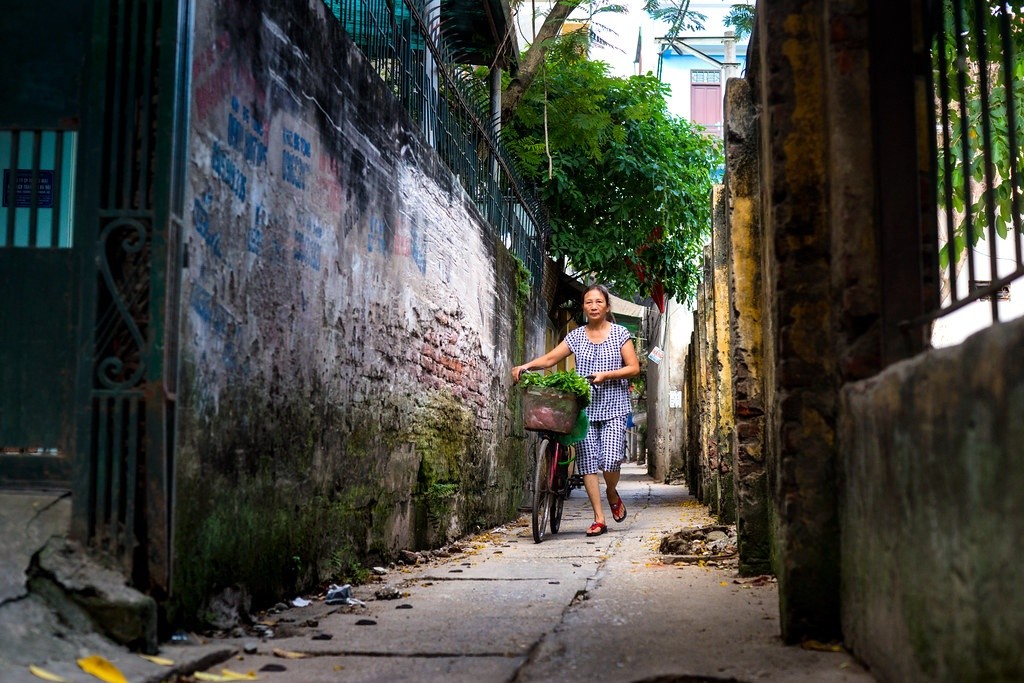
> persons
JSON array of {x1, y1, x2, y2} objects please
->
[{"x1": 512, "y1": 285, "x2": 640, "y2": 534}]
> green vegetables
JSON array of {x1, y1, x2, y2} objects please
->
[{"x1": 519, "y1": 367, "x2": 591, "y2": 409}]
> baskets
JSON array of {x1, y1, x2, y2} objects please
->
[{"x1": 522, "y1": 385, "x2": 579, "y2": 432}]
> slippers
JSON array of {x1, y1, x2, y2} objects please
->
[
  {"x1": 606, "y1": 488, "x2": 627, "y2": 523},
  {"x1": 587, "y1": 521, "x2": 607, "y2": 536}
]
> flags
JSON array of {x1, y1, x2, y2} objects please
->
[{"x1": 627, "y1": 226, "x2": 665, "y2": 313}]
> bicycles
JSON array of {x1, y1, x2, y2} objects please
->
[{"x1": 511, "y1": 364, "x2": 597, "y2": 545}]
